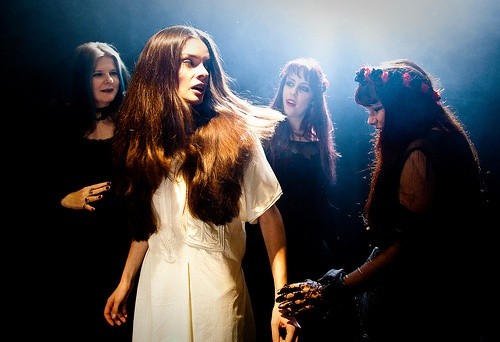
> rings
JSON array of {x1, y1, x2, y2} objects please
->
[
  {"x1": 83, "y1": 203, "x2": 87, "y2": 208},
  {"x1": 89, "y1": 189, "x2": 93, "y2": 196},
  {"x1": 85, "y1": 198, "x2": 89, "y2": 203}
]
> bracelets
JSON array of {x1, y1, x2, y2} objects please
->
[{"x1": 345, "y1": 260, "x2": 377, "y2": 283}]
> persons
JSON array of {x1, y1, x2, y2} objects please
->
[
  {"x1": 42, "y1": 41, "x2": 157, "y2": 342},
  {"x1": 276, "y1": 58, "x2": 500, "y2": 342},
  {"x1": 103, "y1": 25, "x2": 302, "y2": 342},
  {"x1": 244, "y1": 58, "x2": 338, "y2": 342}
]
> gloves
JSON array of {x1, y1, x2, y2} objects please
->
[{"x1": 277, "y1": 269, "x2": 346, "y2": 320}]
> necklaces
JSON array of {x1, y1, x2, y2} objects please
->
[
  {"x1": 95, "y1": 103, "x2": 120, "y2": 121},
  {"x1": 294, "y1": 131, "x2": 304, "y2": 137}
]
